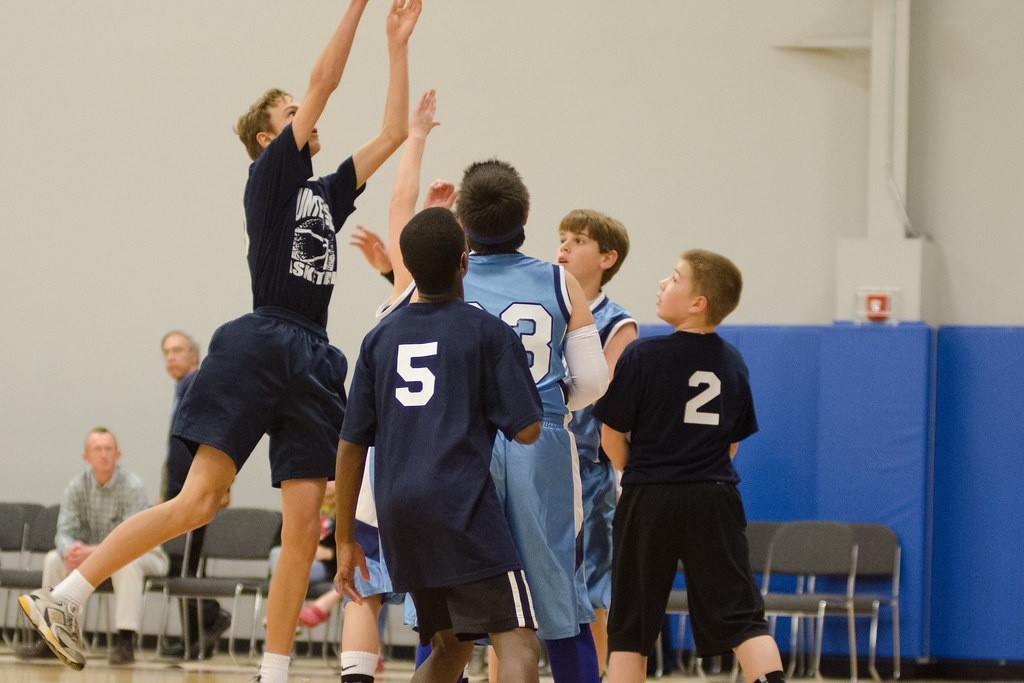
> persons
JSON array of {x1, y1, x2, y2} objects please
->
[
  {"x1": 600, "y1": 247, "x2": 797, "y2": 683},
  {"x1": 267, "y1": 479, "x2": 389, "y2": 673},
  {"x1": 17, "y1": 0, "x2": 422, "y2": 683},
  {"x1": 159, "y1": 328, "x2": 233, "y2": 660},
  {"x1": 14, "y1": 428, "x2": 171, "y2": 665},
  {"x1": 334, "y1": 87, "x2": 640, "y2": 683}
]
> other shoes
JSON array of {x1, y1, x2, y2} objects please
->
[
  {"x1": 299, "y1": 602, "x2": 332, "y2": 628},
  {"x1": 185, "y1": 609, "x2": 231, "y2": 659},
  {"x1": 109, "y1": 640, "x2": 135, "y2": 665},
  {"x1": 17, "y1": 640, "x2": 59, "y2": 659},
  {"x1": 374, "y1": 658, "x2": 384, "y2": 673}
]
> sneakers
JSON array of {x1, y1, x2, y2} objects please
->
[{"x1": 18, "y1": 586, "x2": 87, "y2": 671}]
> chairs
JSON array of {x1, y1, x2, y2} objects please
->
[
  {"x1": 0, "y1": 502, "x2": 392, "y2": 671},
  {"x1": 655, "y1": 522, "x2": 902, "y2": 682}
]
